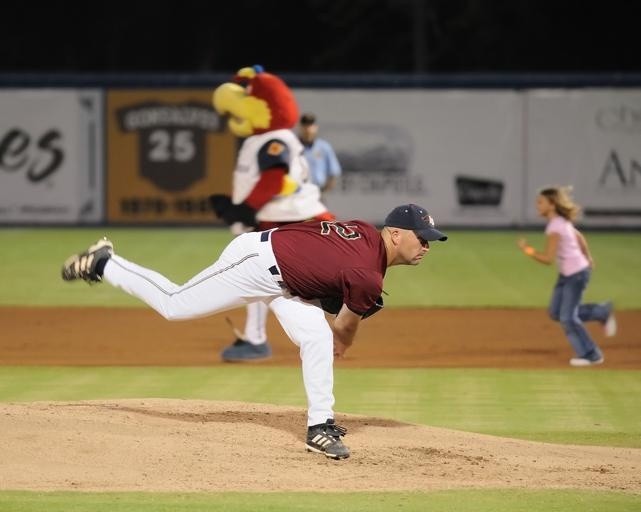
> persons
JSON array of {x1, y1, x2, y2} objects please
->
[
  {"x1": 291, "y1": 112, "x2": 340, "y2": 196},
  {"x1": 514, "y1": 185, "x2": 617, "y2": 368},
  {"x1": 59, "y1": 202, "x2": 448, "y2": 460}
]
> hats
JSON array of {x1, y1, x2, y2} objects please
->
[{"x1": 385, "y1": 204, "x2": 447, "y2": 241}]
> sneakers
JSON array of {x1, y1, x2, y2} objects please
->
[
  {"x1": 60, "y1": 237, "x2": 113, "y2": 282},
  {"x1": 569, "y1": 357, "x2": 604, "y2": 366},
  {"x1": 304, "y1": 419, "x2": 349, "y2": 459},
  {"x1": 604, "y1": 316, "x2": 615, "y2": 337}
]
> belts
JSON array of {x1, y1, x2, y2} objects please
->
[{"x1": 261, "y1": 232, "x2": 286, "y2": 289}]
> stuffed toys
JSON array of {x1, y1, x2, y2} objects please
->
[{"x1": 203, "y1": 62, "x2": 342, "y2": 361}]
